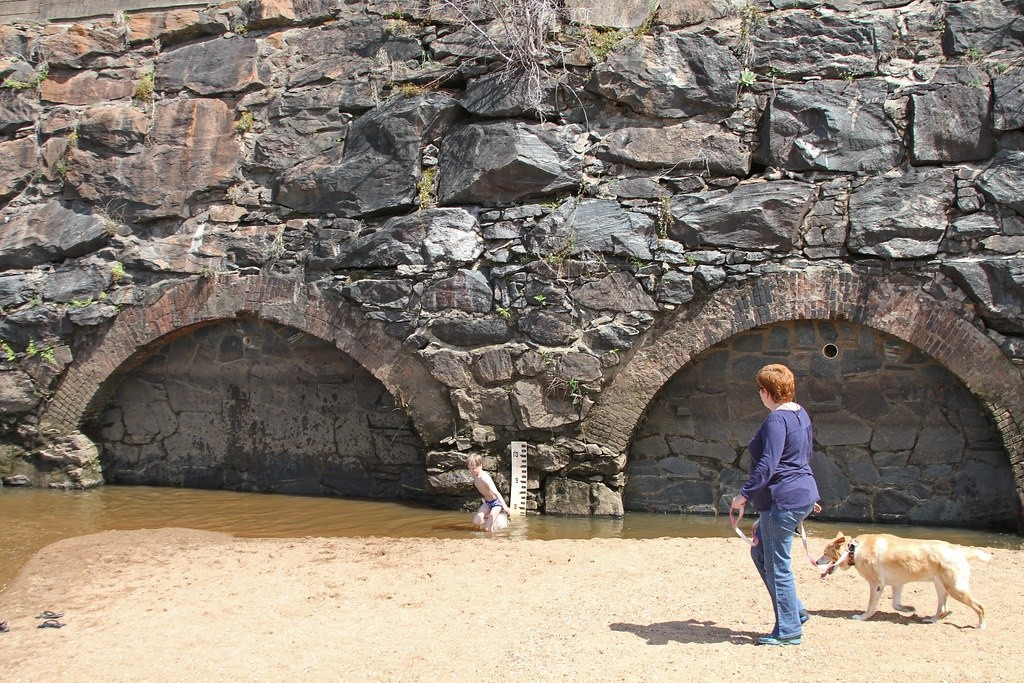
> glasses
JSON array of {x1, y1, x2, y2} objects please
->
[{"x1": 757, "y1": 387, "x2": 768, "y2": 392}]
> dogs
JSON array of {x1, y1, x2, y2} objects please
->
[{"x1": 814, "y1": 530, "x2": 992, "y2": 629}]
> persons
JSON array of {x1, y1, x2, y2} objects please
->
[
  {"x1": 466, "y1": 453, "x2": 510, "y2": 532},
  {"x1": 729, "y1": 363, "x2": 821, "y2": 646}
]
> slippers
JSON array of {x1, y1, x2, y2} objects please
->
[
  {"x1": 36, "y1": 611, "x2": 63, "y2": 618},
  {"x1": 38, "y1": 620, "x2": 65, "y2": 628}
]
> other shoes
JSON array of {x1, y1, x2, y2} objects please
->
[
  {"x1": 0, "y1": 621, "x2": 8, "y2": 632},
  {"x1": 758, "y1": 637, "x2": 801, "y2": 645},
  {"x1": 799, "y1": 609, "x2": 809, "y2": 624}
]
